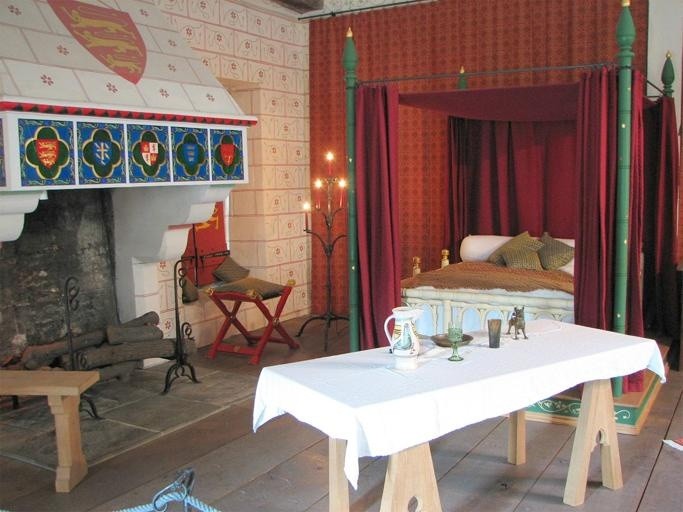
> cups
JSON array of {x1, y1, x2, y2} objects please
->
[{"x1": 488, "y1": 319, "x2": 501, "y2": 348}]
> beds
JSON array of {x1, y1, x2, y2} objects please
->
[{"x1": 343, "y1": 0, "x2": 682, "y2": 399}]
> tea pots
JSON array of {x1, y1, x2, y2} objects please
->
[{"x1": 383, "y1": 307, "x2": 423, "y2": 358}]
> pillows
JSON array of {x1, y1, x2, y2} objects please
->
[
  {"x1": 504, "y1": 250, "x2": 541, "y2": 272},
  {"x1": 181, "y1": 274, "x2": 199, "y2": 301},
  {"x1": 537, "y1": 229, "x2": 573, "y2": 269},
  {"x1": 212, "y1": 255, "x2": 249, "y2": 282},
  {"x1": 487, "y1": 230, "x2": 543, "y2": 266}
]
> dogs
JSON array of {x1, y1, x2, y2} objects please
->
[{"x1": 506, "y1": 306, "x2": 528, "y2": 340}]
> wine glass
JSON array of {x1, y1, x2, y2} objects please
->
[{"x1": 446, "y1": 321, "x2": 463, "y2": 361}]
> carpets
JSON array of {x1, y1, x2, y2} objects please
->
[{"x1": 501, "y1": 335, "x2": 671, "y2": 436}]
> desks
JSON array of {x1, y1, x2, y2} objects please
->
[{"x1": 253, "y1": 320, "x2": 667, "y2": 512}]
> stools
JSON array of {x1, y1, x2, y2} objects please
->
[
  {"x1": 205, "y1": 276, "x2": 301, "y2": 365},
  {"x1": 1, "y1": 369, "x2": 101, "y2": 495}
]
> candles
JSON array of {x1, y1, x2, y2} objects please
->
[{"x1": 300, "y1": 149, "x2": 347, "y2": 232}]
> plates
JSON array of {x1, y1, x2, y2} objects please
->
[{"x1": 431, "y1": 333, "x2": 473, "y2": 347}]
